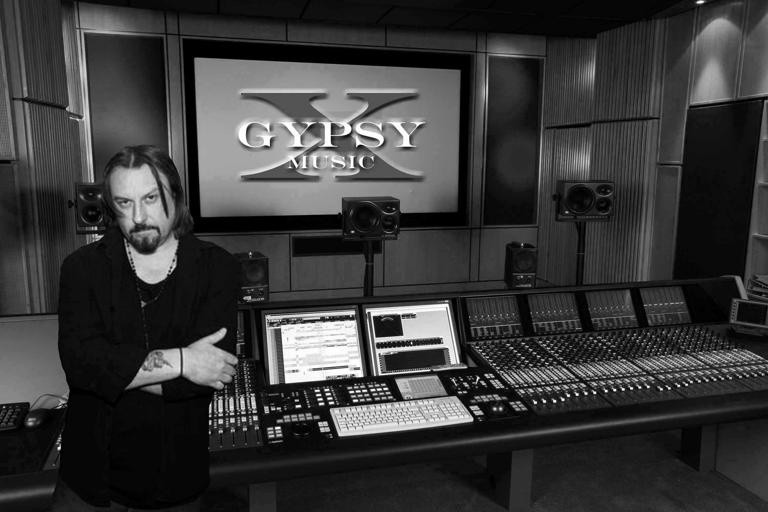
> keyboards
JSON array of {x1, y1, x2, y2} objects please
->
[{"x1": 329, "y1": 396, "x2": 474, "y2": 436}]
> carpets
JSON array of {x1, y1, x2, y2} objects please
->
[{"x1": 202, "y1": 430, "x2": 768, "y2": 512}]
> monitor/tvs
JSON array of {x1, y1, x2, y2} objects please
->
[
  {"x1": 362, "y1": 300, "x2": 463, "y2": 377},
  {"x1": 262, "y1": 305, "x2": 367, "y2": 385}
]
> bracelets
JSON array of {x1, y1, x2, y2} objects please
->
[{"x1": 179, "y1": 347, "x2": 184, "y2": 377}]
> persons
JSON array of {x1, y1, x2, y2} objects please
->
[{"x1": 56, "y1": 143, "x2": 243, "y2": 510}]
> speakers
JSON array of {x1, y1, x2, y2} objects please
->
[
  {"x1": 555, "y1": 180, "x2": 615, "y2": 222},
  {"x1": 75, "y1": 182, "x2": 106, "y2": 235},
  {"x1": 504, "y1": 241, "x2": 538, "y2": 289},
  {"x1": 234, "y1": 252, "x2": 269, "y2": 304},
  {"x1": 342, "y1": 196, "x2": 400, "y2": 241}
]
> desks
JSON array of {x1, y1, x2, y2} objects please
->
[{"x1": 0, "y1": 375, "x2": 768, "y2": 512}]
